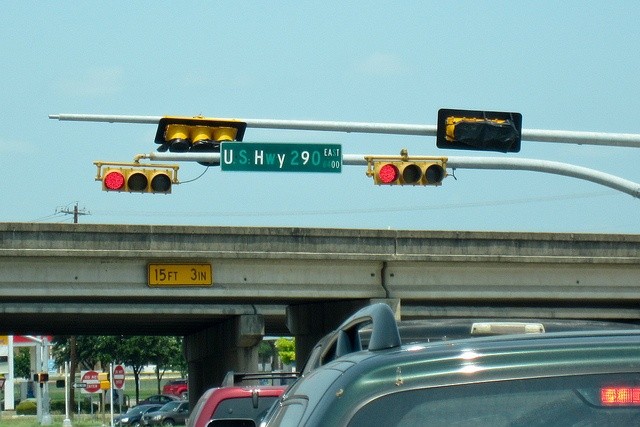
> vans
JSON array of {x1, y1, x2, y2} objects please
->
[{"x1": 189, "y1": 386, "x2": 288, "y2": 427}]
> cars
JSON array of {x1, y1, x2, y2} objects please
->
[
  {"x1": 109, "y1": 404, "x2": 162, "y2": 427},
  {"x1": 164, "y1": 380, "x2": 189, "y2": 398},
  {"x1": 139, "y1": 395, "x2": 180, "y2": 404},
  {"x1": 140, "y1": 400, "x2": 189, "y2": 427}
]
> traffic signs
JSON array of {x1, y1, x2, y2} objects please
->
[
  {"x1": 76, "y1": 383, "x2": 87, "y2": 388},
  {"x1": 221, "y1": 142, "x2": 341, "y2": 172}
]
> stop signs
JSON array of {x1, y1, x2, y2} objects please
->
[
  {"x1": 81, "y1": 371, "x2": 102, "y2": 393},
  {"x1": 113, "y1": 366, "x2": 125, "y2": 388}
]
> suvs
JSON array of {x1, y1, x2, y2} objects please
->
[{"x1": 259, "y1": 304, "x2": 639, "y2": 426}]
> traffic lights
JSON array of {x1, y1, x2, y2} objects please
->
[
  {"x1": 437, "y1": 108, "x2": 522, "y2": 153},
  {"x1": 374, "y1": 159, "x2": 447, "y2": 185},
  {"x1": 155, "y1": 117, "x2": 247, "y2": 152},
  {"x1": 102, "y1": 168, "x2": 172, "y2": 193}
]
